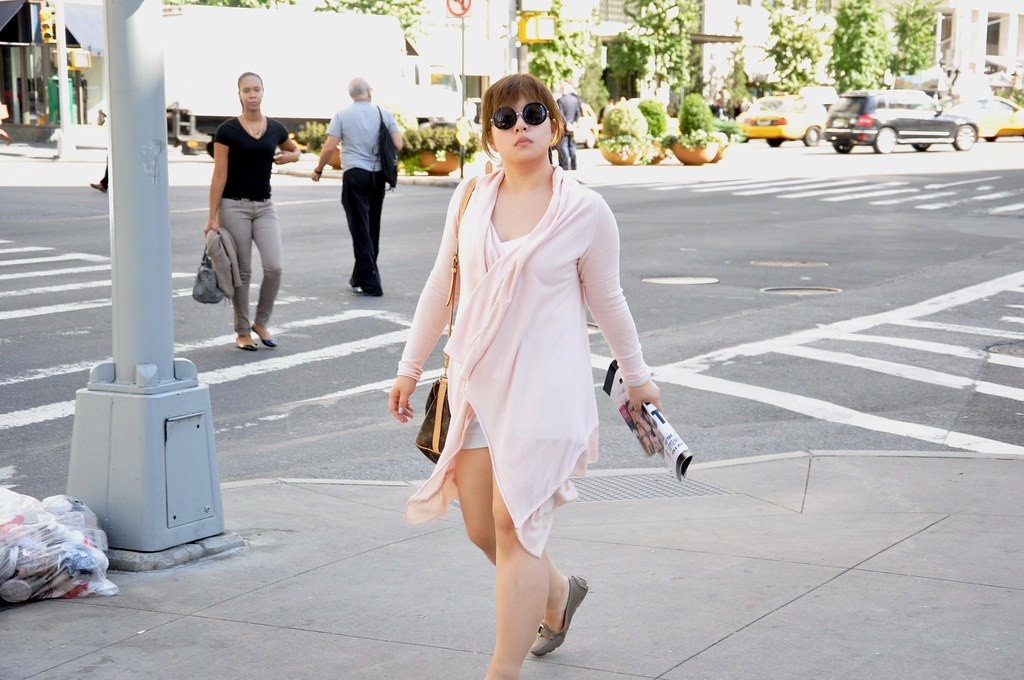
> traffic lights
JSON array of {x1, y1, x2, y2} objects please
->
[
  {"x1": 71, "y1": 50, "x2": 92, "y2": 70},
  {"x1": 39, "y1": 6, "x2": 54, "y2": 44}
]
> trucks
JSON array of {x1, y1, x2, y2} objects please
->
[{"x1": 163, "y1": 4, "x2": 467, "y2": 157}]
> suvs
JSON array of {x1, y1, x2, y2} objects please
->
[{"x1": 823, "y1": 88, "x2": 983, "y2": 154}]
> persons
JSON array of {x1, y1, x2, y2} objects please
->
[
  {"x1": 90, "y1": 154, "x2": 108, "y2": 192},
  {"x1": 390, "y1": 73, "x2": 662, "y2": 680},
  {"x1": 555, "y1": 76, "x2": 585, "y2": 171},
  {"x1": 204, "y1": 72, "x2": 301, "y2": 351},
  {"x1": 310, "y1": 77, "x2": 404, "y2": 297}
]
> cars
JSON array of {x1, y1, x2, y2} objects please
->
[
  {"x1": 936, "y1": 93, "x2": 1024, "y2": 142},
  {"x1": 735, "y1": 94, "x2": 829, "y2": 148}
]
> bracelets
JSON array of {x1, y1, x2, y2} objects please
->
[{"x1": 313, "y1": 167, "x2": 322, "y2": 174}]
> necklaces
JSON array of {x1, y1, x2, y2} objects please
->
[{"x1": 241, "y1": 114, "x2": 263, "y2": 139}]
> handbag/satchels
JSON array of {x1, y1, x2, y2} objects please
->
[
  {"x1": 192, "y1": 245, "x2": 224, "y2": 303},
  {"x1": 416, "y1": 378, "x2": 451, "y2": 465},
  {"x1": 376, "y1": 106, "x2": 399, "y2": 188}
]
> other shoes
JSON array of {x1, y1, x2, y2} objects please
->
[
  {"x1": 91, "y1": 184, "x2": 107, "y2": 192},
  {"x1": 251, "y1": 326, "x2": 277, "y2": 347},
  {"x1": 349, "y1": 278, "x2": 367, "y2": 295},
  {"x1": 530, "y1": 575, "x2": 587, "y2": 657},
  {"x1": 237, "y1": 344, "x2": 257, "y2": 350}
]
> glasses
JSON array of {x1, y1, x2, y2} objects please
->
[{"x1": 491, "y1": 102, "x2": 549, "y2": 131}]
